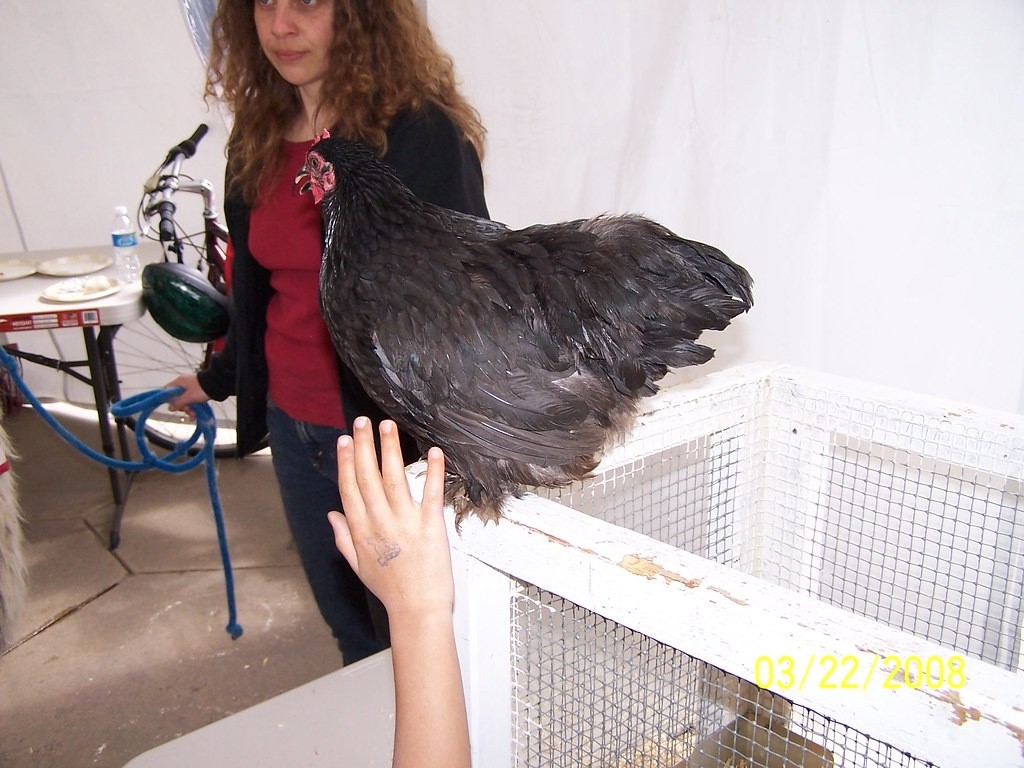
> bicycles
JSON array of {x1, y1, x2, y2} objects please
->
[{"x1": 96, "y1": 123, "x2": 272, "y2": 460}]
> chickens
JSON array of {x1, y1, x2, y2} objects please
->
[{"x1": 295, "y1": 129, "x2": 755, "y2": 532}]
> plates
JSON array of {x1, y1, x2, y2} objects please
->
[
  {"x1": 38, "y1": 255, "x2": 111, "y2": 275},
  {"x1": 41, "y1": 277, "x2": 121, "y2": 301},
  {"x1": 0, "y1": 259, "x2": 36, "y2": 280}
]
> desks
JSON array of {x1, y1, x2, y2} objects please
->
[{"x1": 0, "y1": 243, "x2": 167, "y2": 549}]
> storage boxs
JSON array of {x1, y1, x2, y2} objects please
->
[{"x1": 677, "y1": 712, "x2": 833, "y2": 768}]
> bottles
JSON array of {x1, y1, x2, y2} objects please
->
[{"x1": 112, "y1": 207, "x2": 141, "y2": 283}]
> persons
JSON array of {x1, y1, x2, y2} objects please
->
[
  {"x1": 328, "y1": 414, "x2": 475, "y2": 768},
  {"x1": 163, "y1": 0, "x2": 493, "y2": 665}
]
